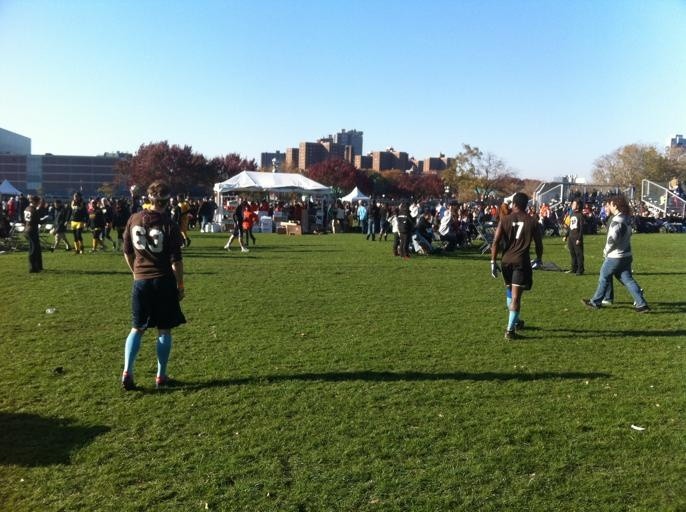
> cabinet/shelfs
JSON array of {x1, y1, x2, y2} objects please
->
[{"x1": 221, "y1": 195, "x2": 241, "y2": 224}]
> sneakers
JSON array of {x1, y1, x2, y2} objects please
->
[
  {"x1": 530, "y1": 258, "x2": 543, "y2": 270},
  {"x1": 580, "y1": 297, "x2": 600, "y2": 310},
  {"x1": 29, "y1": 265, "x2": 44, "y2": 273},
  {"x1": 634, "y1": 305, "x2": 650, "y2": 314},
  {"x1": 121, "y1": 371, "x2": 134, "y2": 389},
  {"x1": 156, "y1": 376, "x2": 170, "y2": 388},
  {"x1": 180, "y1": 239, "x2": 191, "y2": 249},
  {"x1": 241, "y1": 247, "x2": 250, "y2": 253},
  {"x1": 504, "y1": 328, "x2": 516, "y2": 340},
  {"x1": 564, "y1": 269, "x2": 583, "y2": 276},
  {"x1": 515, "y1": 319, "x2": 525, "y2": 331},
  {"x1": 223, "y1": 245, "x2": 231, "y2": 252},
  {"x1": 366, "y1": 233, "x2": 388, "y2": 241},
  {"x1": 50, "y1": 241, "x2": 116, "y2": 254},
  {"x1": 393, "y1": 251, "x2": 411, "y2": 259}
]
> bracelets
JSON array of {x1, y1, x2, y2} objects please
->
[{"x1": 177, "y1": 282, "x2": 183, "y2": 288}]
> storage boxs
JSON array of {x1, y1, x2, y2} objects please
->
[
  {"x1": 252, "y1": 216, "x2": 272, "y2": 233},
  {"x1": 280, "y1": 221, "x2": 302, "y2": 236},
  {"x1": 225, "y1": 224, "x2": 234, "y2": 231}
]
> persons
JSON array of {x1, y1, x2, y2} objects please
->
[
  {"x1": 23, "y1": 196, "x2": 54, "y2": 272},
  {"x1": 532, "y1": 203, "x2": 571, "y2": 241},
  {"x1": 490, "y1": 193, "x2": 543, "y2": 338},
  {"x1": 251, "y1": 199, "x2": 300, "y2": 219},
  {"x1": 581, "y1": 195, "x2": 650, "y2": 313},
  {"x1": 388, "y1": 198, "x2": 512, "y2": 258},
  {"x1": 633, "y1": 208, "x2": 686, "y2": 233},
  {"x1": 42, "y1": 192, "x2": 149, "y2": 253},
  {"x1": 562, "y1": 199, "x2": 586, "y2": 276},
  {"x1": 600, "y1": 200, "x2": 645, "y2": 305},
  {"x1": 121, "y1": 180, "x2": 186, "y2": 389},
  {"x1": 337, "y1": 198, "x2": 390, "y2": 241},
  {"x1": 224, "y1": 197, "x2": 259, "y2": 253},
  {"x1": 569, "y1": 190, "x2": 615, "y2": 234},
  {"x1": 170, "y1": 194, "x2": 219, "y2": 248},
  {"x1": 0, "y1": 193, "x2": 31, "y2": 239}
]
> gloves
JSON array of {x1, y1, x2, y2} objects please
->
[
  {"x1": 490, "y1": 259, "x2": 502, "y2": 280},
  {"x1": 177, "y1": 287, "x2": 184, "y2": 303}
]
